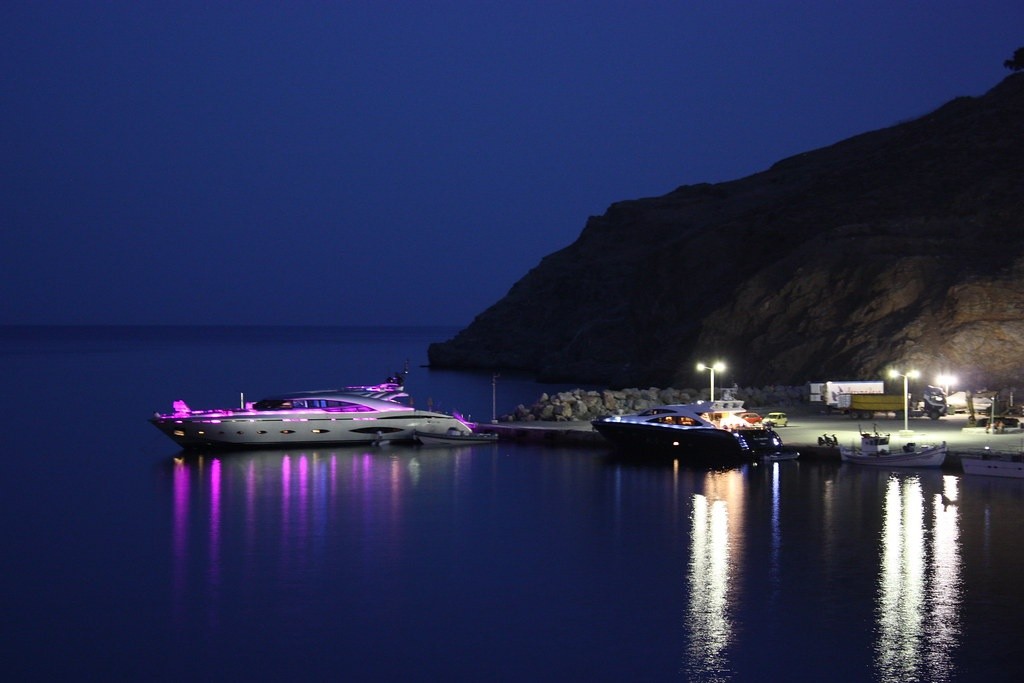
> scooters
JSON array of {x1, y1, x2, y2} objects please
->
[{"x1": 818, "y1": 433, "x2": 838, "y2": 447}]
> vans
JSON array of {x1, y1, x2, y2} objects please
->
[{"x1": 762, "y1": 413, "x2": 788, "y2": 427}]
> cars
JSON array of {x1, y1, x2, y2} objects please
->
[
  {"x1": 986, "y1": 417, "x2": 1004, "y2": 427},
  {"x1": 997, "y1": 419, "x2": 1024, "y2": 428},
  {"x1": 739, "y1": 413, "x2": 762, "y2": 426},
  {"x1": 978, "y1": 418, "x2": 988, "y2": 427},
  {"x1": 1008, "y1": 409, "x2": 1024, "y2": 417}
]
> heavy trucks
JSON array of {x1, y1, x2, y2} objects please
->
[{"x1": 836, "y1": 386, "x2": 948, "y2": 420}]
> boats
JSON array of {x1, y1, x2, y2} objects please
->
[
  {"x1": 960, "y1": 449, "x2": 1024, "y2": 478},
  {"x1": 839, "y1": 432, "x2": 947, "y2": 466},
  {"x1": 372, "y1": 440, "x2": 390, "y2": 446},
  {"x1": 147, "y1": 370, "x2": 473, "y2": 451},
  {"x1": 412, "y1": 428, "x2": 499, "y2": 446},
  {"x1": 590, "y1": 399, "x2": 783, "y2": 467}
]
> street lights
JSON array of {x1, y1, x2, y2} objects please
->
[
  {"x1": 698, "y1": 363, "x2": 722, "y2": 402},
  {"x1": 890, "y1": 371, "x2": 918, "y2": 430}
]
